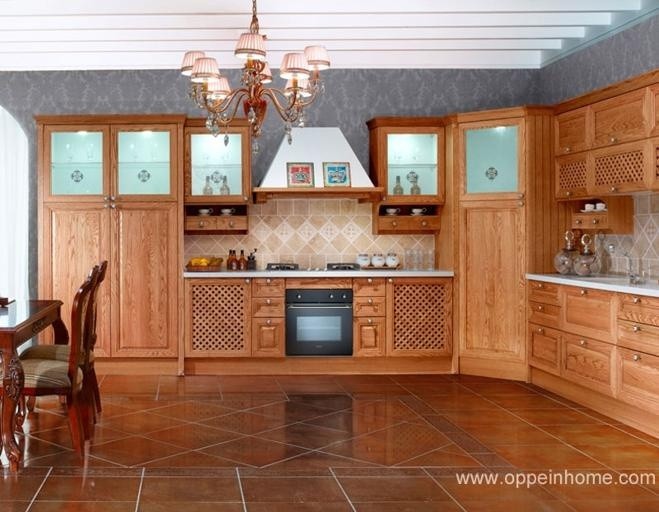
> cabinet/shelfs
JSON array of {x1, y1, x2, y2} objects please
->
[
  {"x1": 387, "y1": 277, "x2": 452, "y2": 376},
  {"x1": 372, "y1": 204, "x2": 441, "y2": 235},
  {"x1": 182, "y1": 277, "x2": 251, "y2": 376},
  {"x1": 366, "y1": 115, "x2": 447, "y2": 205},
  {"x1": 353, "y1": 278, "x2": 386, "y2": 375},
  {"x1": 250, "y1": 278, "x2": 287, "y2": 377},
  {"x1": 552, "y1": 68, "x2": 659, "y2": 201},
  {"x1": 36, "y1": 113, "x2": 183, "y2": 199},
  {"x1": 567, "y1": 195, "x2": 635, "y2": 238},
  {"x1": 613, "y1": 293, "x2": 659, "y2": 440},
  {"x1": 561, "y1": 284, "x2": 614, "y2": 419},
  {"x1": 445, "y1": 105, "x2": 572, "y2": 385},
  {"x1": 527, "y1": 280, "x2": 560, "y2": 395},
  {"x1": 37, "y1": 200, "x2": 183, "y2": 375},
  {"x1": 184, "y1": 202, "x2": 252, "y2": 237},
  {"x1": 183, "y1": 115, "x2": 254, "y2": 205}
]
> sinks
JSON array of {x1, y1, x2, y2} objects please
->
[{"x1": 573, "y1": 274, "x2": 630, "y2": 287}]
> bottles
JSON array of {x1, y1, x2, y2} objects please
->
[
  {"x1": 227, "y1": 248, "x2": 247, "y2": 272},
  {"x1": 394, "y1": 175, "x2": 403, "y2": 196},
  {"x1": 410, "y1": 174, "x2": 421, "y2": 195},
  {"x1": 203, "y1": 176, "x2": 213, "y2": 195},
  {"x1": 220, "y1": 176, "x2": 230, "y2": 195},
  {"x1": 246, "y1": 252, "x2": 257, "y2": 271}
]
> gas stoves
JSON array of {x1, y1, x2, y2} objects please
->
[{"x1": 266, "y1": 262, "x2": 360, "y2": 271}]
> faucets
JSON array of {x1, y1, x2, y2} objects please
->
[{"x1": 621, "y1": 248, "x2": 636, "y2": 280}]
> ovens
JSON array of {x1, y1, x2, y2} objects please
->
[{"x1": 285, "y1": 288, "x2": 353, "y2": 356}]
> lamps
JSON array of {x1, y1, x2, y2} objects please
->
[{"x1": 176, "y1": 0, "x2": 332, "y2": 146}]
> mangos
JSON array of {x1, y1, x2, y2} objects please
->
[{"x1": 190, "y1": 258, "x2": 223, "y2": 266}]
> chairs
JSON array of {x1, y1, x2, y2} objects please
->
[
  {"x1": 0, "y1": 263, "x2": 100, "y2": 458},
  {"x1": 14, "y1": 258, "x2": 109, "y2": 431}
]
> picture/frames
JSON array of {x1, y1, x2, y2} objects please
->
[
  {"x1": 286, "y1": 162, "x2": 315, "y2": 188},
  {"x1": 322, "y1": 162, "x2": 351, "y2": 189}
]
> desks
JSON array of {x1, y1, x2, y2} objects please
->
[{"x1": 0, "y1": 299, "x2": 70, "y2": 474}]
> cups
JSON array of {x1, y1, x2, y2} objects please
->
[
  {"x1": 198, "y1": 208, "x2": 213, "y2": 213},
  {"x1": 596, "y1": 203, "x2": 606, "y2": 210},
  {"x1": 584, "y1": 203, "x2": 595, "y2": 210},
  {"x1": 221, "y1": 208, "x2": 236, "y2": 213},
  {"x1": 411, "y1": 208, "x2": 428, "y2": 213},
  {"x1": 405, "y1": 248, "x2": 436, "y2": 272},
  {"x1": 385, "y1": 207, "x2": 401, "y2": 213}
]
